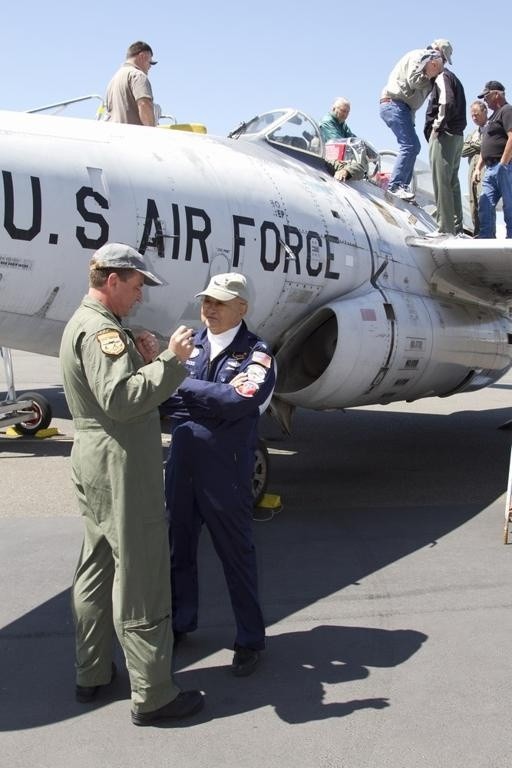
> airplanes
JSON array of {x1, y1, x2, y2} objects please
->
[{"x1": 0, "y1": 94, "x2": 512, "y2": 512}]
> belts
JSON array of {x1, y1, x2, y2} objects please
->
[{"x1": 378, "y1": 97, "x2": 396, "y2": 103}]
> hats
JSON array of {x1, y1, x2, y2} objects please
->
[
  {"x1": 89, "y1": 241, "x2": 162, "y2": 286},
  {"x1": 194, "y1": 273, "x2": 250, "y2": 305},
  {"x1": 413, "y1": 38, "x2": 453, "y2": 75},
  {"x1": 477, "y1": 80, "x2": 504, "y2": 99}
]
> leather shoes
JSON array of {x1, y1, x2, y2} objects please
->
[
  {"x1": 232, "y1": 644, "x2": 260, "y2": 676},
  {"x1": 76, "y1": 660, "x2": 117, "y2": 702},
  {"x1": 130, "y1": 690, "x2": 204, "y2": 726}
]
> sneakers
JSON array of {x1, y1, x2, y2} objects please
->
[
  {"x1": 389, "y1": 185, "x2": 416, "y2": 204},
  {"x1": 425, "y1": 229, "x2": 464, "y2": 240}
]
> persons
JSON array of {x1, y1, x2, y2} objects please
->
[
  {"x1": 157, "y1": 273, "x2": 277, "y2": 676},
  {"x1": 59, "y1": 243, "x2": 204, "y2": 726},
  {"x1": 107, "y1": 42, "x2": 156, "y2": 126},
  {"x1": 316, "y1": 39, "x2": 512, "y2": 238}
]
